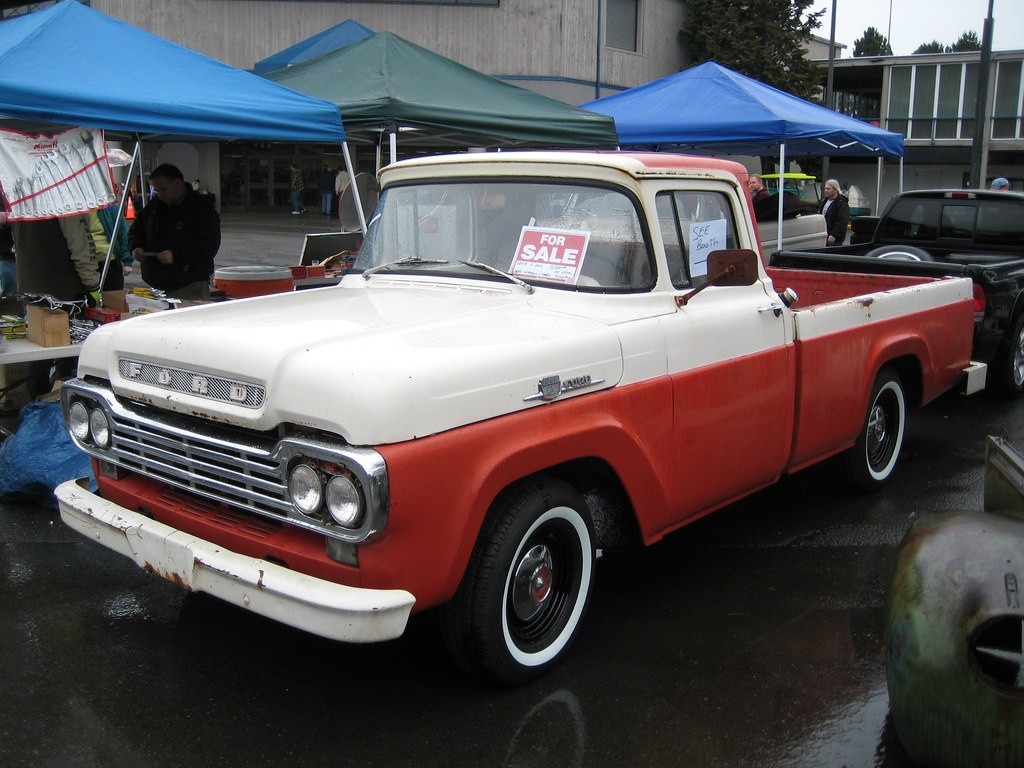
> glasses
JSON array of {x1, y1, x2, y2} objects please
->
[{"x1": 749, "y1": 182, "x2": 756, "y2": 184}]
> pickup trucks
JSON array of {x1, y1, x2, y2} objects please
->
[
  {"x1": 769, "y1": 177, "x2": 1022, "y2": 407},
  {"x1": 52, "y1": 149, "x2": 989, "y2": 689}
]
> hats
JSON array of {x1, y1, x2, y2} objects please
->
[
  {"x1": 990, "y1": 177, "x2": 1009, "y2": 189},
  {"x1": 825, "y1": 178, "x2": 842, "y2": 194},
  {"x1": 841, "y1": 182, "x2": 848, "y2": 190}
]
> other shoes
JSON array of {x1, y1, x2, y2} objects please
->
[
  {"x1": 301, "y1": 210, "x2": 304, "y2": 213},
  {"x1": 292, "y1": 211, "x2": 300, "y2": 214},
  {"x1": 304, "y1": 207, "x2": 306, "y2": 211}
]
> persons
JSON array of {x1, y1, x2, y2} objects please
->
[
  {"x1": 11, "y1": 213, "x2": 100, "y2": 320},
  {"x1": 989, "y1": 178, "x2": 1008, "y2": 192},
  {"x1": 319, "y1": 162, "x2": 348, "y2": 214},
  {"x1": 748, "y1": 174, "x2": 770, "y2": 202},
  {"x1": 290, "y1": 164, "x2": 304, "y2": 214},
  {"x1": 88, "y1": 204, "x2": 134, "y2": 291},
  {"x1": 0, "y1": 192, "x2": 18, "y2": 291},
  {"x1": 136, "y1": 170, "x2": 151, "y2": 207},
  {"x1": 819, "y1": 179, "x2": 850, "y2": 247},
  {"x1": 127, "y1": 163, "x2": 222, "y2": 300}
]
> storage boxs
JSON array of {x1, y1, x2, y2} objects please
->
[
  {"x1": 0, "y1": 361, "x2": 34, "y2": 414},
  {"x1": 100, "y1": 289, "x2": 133, "y2": 312},
  {"x1": 23, "y1": 304, "x2": 70, "y2": 347}
]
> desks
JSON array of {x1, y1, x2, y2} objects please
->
[
  {"x1": 291, "y1": 272, "x2": 344, "y2": 291},
  {"x1": 0, "y1": 328, "x2": 86, "y2": 374}
]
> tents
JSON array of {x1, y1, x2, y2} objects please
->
[
  {"x1": 578, "y1": 62, "x2": 904, "y2": 249},
  {"x1": 262, "y1": 31, "x2": 621, "y2": 200},
  {"x1": 246, "y1": 19, "x2": 377, "y2": 76},
  {"x1": 0, "y1": 0, "x2": 368, "y2": 237}
]
